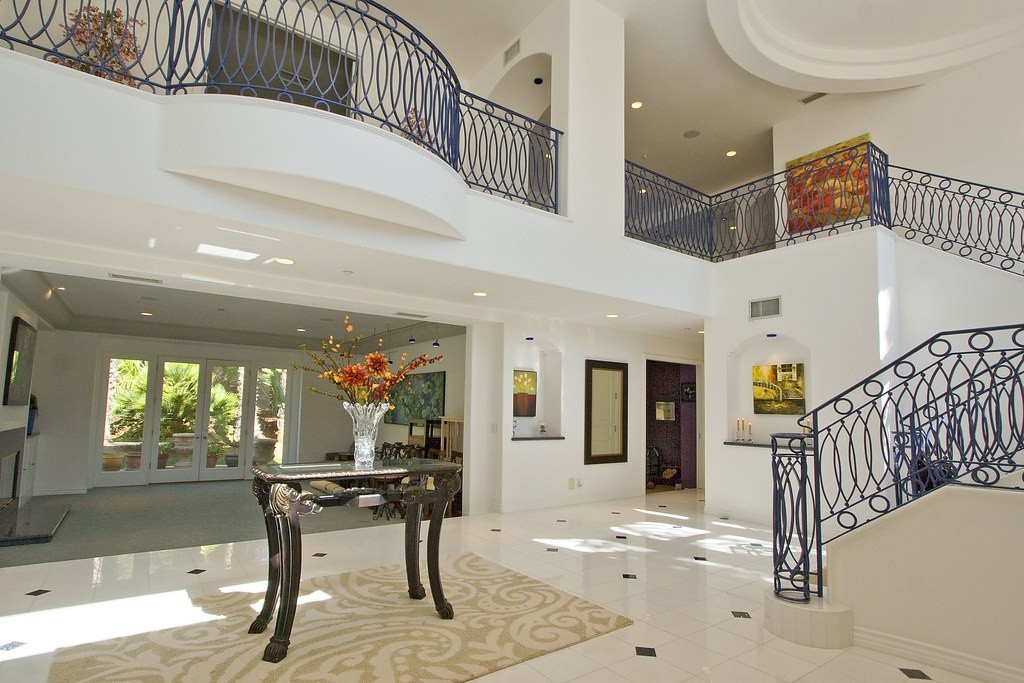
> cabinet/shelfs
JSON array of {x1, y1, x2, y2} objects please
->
[{"x1": 19, "y1": 435, "x2": 40, "y2": 509}]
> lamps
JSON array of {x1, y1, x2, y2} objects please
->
[
  {"x1": 388, "y1": 330, "x2": 393, "y2": 365},
  {"x1": 432, "y1": 323, "x2": 441, "y2": 348},
  {"x1": 409, "y1": 321, "x2": 415, "y2": 344}
]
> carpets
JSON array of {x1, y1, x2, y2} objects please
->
[{"x1": 8, "y1": 551, "x2": 638, "y2": 683}]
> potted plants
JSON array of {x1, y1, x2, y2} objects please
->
[
  {"x1": 206, "y1": 445, "x2": 222, "y2": 467},
  {"x1": 157, "y1": 426, "x2": 175, "y2": 469}
]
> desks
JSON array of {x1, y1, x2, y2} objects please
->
[{"x1": 247, "y1": 458, "x2": 465, "y2": 665}]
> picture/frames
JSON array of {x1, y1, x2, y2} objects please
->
[
  {"x1": 383, "y1": 371, "x2": 449, "y2": 425},
  {"x1": 656, "y1": 401, "x2": 676, "y2": 421}
]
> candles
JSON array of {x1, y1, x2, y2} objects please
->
[
  {"x1": 748, "y1": 423, "x2": 752, "y2": 435},
  {"x1": 807, "y1": 418, "x2": 811, "y2": 432},
  {"x1": 801, "y1": 424, "x2": 805, "y2": 433},
  {"x1": 735, "y1": 417, "x2": 740, "y2": 431},
  {"x1": 742, "y1": 418, "x2": 745, "y2": 431}
]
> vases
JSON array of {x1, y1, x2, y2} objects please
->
[
  {"x1": 343, "y1": 400, "x2": 389, "y2": 470},
  {"x1": 226, "y1": 455, "x2": 238, "y2": 466},
  {"x1": 103, "y1": 454, "x2": 122, "y2": 471},
  {"x1": 124, "y1": 452, "x2": 141, "y2": 470}
]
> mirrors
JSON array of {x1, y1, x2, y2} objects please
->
[{"x1": 583, "y1": 359, "x2": 629, "y2": 466}]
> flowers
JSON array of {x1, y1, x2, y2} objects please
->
[{"x1": 290, "y1": 314, "x2": 445, "y2": 404}]
